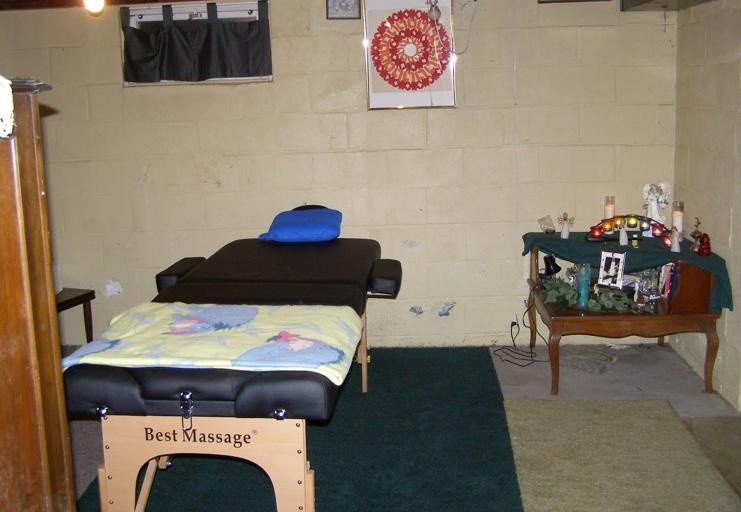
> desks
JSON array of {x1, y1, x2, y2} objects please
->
[{"x1": 526, "y1": 232, "x2": 720, "y2": 396}]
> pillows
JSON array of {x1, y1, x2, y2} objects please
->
[{"x1": 257, "y1": 209, "x2": 344, "y2": 248}]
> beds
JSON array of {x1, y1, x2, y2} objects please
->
[{"x1": 63, "y1": 206, "x2": 402, "y2": 442}]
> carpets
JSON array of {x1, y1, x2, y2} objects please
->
[{"x1": 74, "y1": 347, "x2": 525, "y2": 512}]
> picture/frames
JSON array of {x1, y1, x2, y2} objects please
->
[
  {"x1": 597, "y1": 249, "x2": 627, "y2": 291},
  {"x1": 326, "y1": 0, "x2": 460, "y2": 111}
]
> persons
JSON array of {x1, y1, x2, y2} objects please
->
[{"x1": 603, "y1": 258, "x2": 619, "y2": 283}]
